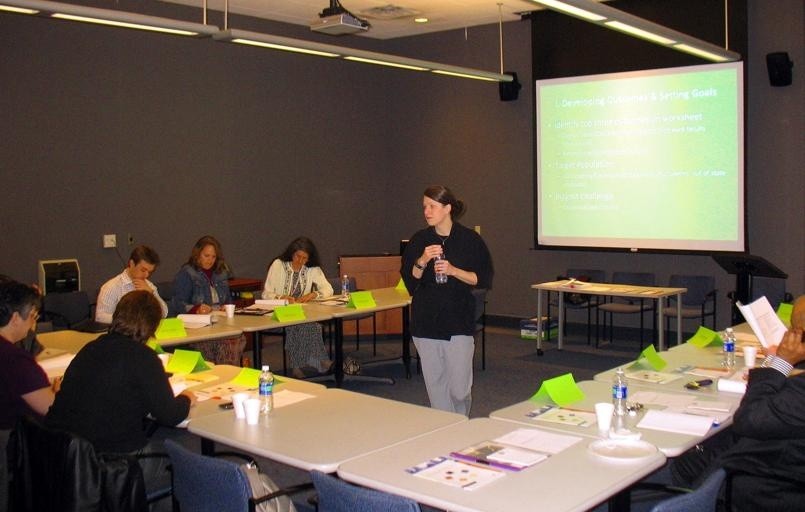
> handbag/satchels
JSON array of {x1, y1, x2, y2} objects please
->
[
  {"x1": 241, "y1": 460, "x2": 298, "y2": 512},
  {"x1": 342, "y1": 358, "x2": 360, "y2": 376}
]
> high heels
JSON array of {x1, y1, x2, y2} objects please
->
[
  {"x1": 320, "y1": 359, "x2": 335, "y2": 372},
  {"x1": 291, "y1": 368, "x2": 305, "y2": 379}
]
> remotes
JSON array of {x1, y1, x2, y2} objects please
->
[{"x1": 686, "y1": 379, "x2": 713, "y2": 389}]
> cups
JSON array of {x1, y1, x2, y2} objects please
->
[
  {"x1": 595, "y1": 402, "x2": 615, "y2": 431},
  {"x1": 157, "y1": 352, "x2": 170, "y2": 368},
  {"x1": 231, "y1": 392, "x2": 248, "y2": 419},
  {"x1": 741, "y1": 345, "x2": 757, "y2": 367},
  {"x1": 224, "y1": 303, "x2": 236, "y2": 319},
  {"x1": 242, "y1": 398, "x2": 261, "y2": 426}
]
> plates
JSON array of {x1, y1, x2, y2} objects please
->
[{"x1": 590, "y1": 439, "x2": 658, "y2": 461}]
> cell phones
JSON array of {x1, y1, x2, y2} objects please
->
[{"x1": 218, "y1": 403, "x2": 236, "y2": 410}]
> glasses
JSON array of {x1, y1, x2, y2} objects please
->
[{"x1": 30, "y1": 311, "x2": 40, "y2": 321}]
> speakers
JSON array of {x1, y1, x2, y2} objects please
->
[
  {"x1": 499, "y1": 72, "x2": 518, "y2": 101},
  {"x1": 766, "y1": 53, "x2": 792, "y2": 86}
]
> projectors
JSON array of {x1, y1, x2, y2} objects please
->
[{"x1": 310, "y1": 14, "x2": 368, "y2": 36}]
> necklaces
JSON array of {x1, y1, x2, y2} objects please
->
[{"x1": 434, "y1": 229, "x2": 450, "y2": 246}]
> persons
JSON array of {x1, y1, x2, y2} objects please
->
[
  {"x1": 94, "y1": 246, "x2": 169, "y2": 324},
  {"x1": 261, "y1": 237, "x2": 334, "y2": 379},
  {"x1": 0, "y1": 274, "x2": 197, "y2": 499},
  {"x1": 400, "y1": 184, "x2": 494, "y2": 416},
  {"x1": 636, "y1": 294, "x2": 804, "y2": 512},
  {"x1": 171, "y1": 236, "x2": 247, "y2": 367}
]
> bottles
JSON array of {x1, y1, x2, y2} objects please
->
[
  {"x1": 723, "y1": 327, "x2": 735, "y2": 367},
  {"x1": 341, "y1": 274, "x2": 350, "y2": 298},
  {"x1": 613, "y1": 366, "x2": 628, "y2": 416},
  {"x1": 433, "y1": 253, "x2": 449, "y2": 285},
  {"x1": 258, "y1": 364, "x2": 274, "y2": 412}
]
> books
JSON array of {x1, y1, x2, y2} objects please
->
[
  {"x1": 450, "y1": 439, "x2": 551, "y2": 472},
  {"x1": 244, "y1": 299, "x2": 289, "y2": 310},
  {"x1": 176, "y1": 314, "x2": 220, "y2": 329}
]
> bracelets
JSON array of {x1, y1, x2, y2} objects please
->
[{"x1": 415, "y1": 259, "x2": 427, "y2": 270}]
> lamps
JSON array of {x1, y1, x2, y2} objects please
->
[
  {"x1": 0, "y1": 0, "x2": 219, "y2": 38},
  {"x1": 523, "y1": 0, "x2": 740, "y2": 62},
  {"x1": 212, "y1": 0, "x2": 513, "y2": 82}
]
